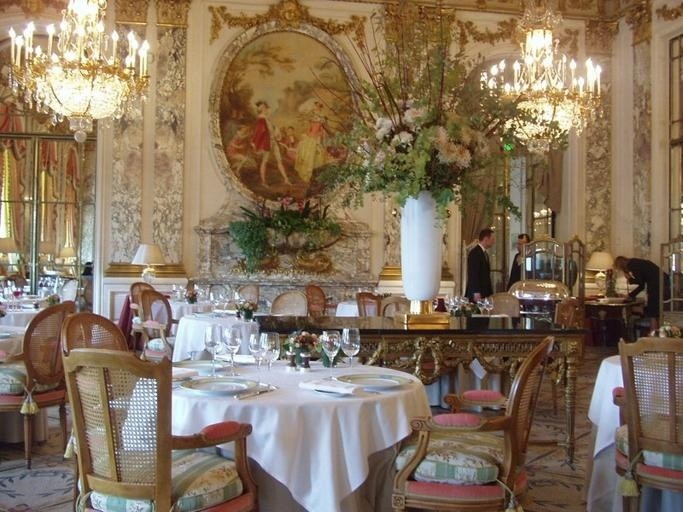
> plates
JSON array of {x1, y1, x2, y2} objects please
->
[
  {"x1": 179, "y1": 377, "x2": 259, "y2": 394},
  {"x1": 0, "y1": 332, "x2": 10, "y2": 338},
  {"x1": 336, "y1": 373, "x2": 415, "y2": 389},
  {"x1": 597, "y1": 297, "x2": 626, "y2": 304}
]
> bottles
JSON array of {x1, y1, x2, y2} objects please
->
[
  {"x1": 448, "y1": 306, "x2": 467, "y2": 330},
  {"x1": 287, "y1": 351, "x2": 311, "y2": 369},
  {"x1": 39, "y1": 276, "x2": 61, "y2": 300}
]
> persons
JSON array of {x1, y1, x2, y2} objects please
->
[
  {"x1": 613, "y1": 256, "x2": 670, "y2": 336},
  {"x1": 507, "y1": 233, "x2": 530, "y2": 292},
  {"x1": 465, "y1": 228, "x2": 495, "y2": 335},
  {"x1": 227, "y1": 99, "x2": 350, "y2": 188},
  {"x1": 81, "y1": 262, "x2": 92, "y2": 275}
]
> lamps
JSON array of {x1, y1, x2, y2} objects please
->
[
  {"x1": 131, "y1": 244, "x2": 166, "y2": 283},
  {"x1": 0, "y1": 237, "x2": 18, "y2": 274},
  {"x1": 59, "y1": 247, "x2": 77, "y2": 274},
  {"x1": 38, "y1": 240, "x2": 55, "y2": 260},
  {"x1": 584, "y1": 252, "x2": 613, "y2": 290},
  {"x1": 9, "y1": 0, "x2": 151, "y2": 143},
  {"x1": 481, "y1": 1, "x2": 602, "y2": 160}
]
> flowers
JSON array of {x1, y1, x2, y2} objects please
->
[{"x1": 314, "y1": 6, "x2": 569, "y2": 222}]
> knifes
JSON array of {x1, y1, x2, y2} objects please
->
[{"x1": 313, "y1": 389, "x2": 380, "y2": 396}]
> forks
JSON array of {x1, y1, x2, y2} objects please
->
[{"x1": 233, "y1": 383, "x2": 280, "y2": 399}]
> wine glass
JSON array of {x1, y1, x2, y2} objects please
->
[
  {"x1": 2, "y1": 280, "x2": 25, "y2": 312},
  {"x1": 173, "y1": 283, "x2": 207, "y2": 304},
  {"x1": 322, "y1": 328, "x2": 361, "y2": 375},
  {"x1": 204, "y1": 326, "x2": 281, "y2": 389},
  {"x1": 208, "y1": 289, "x2": 240, "y2": 318},
  {"x1": 430, "y1": 297, "x2": 493, "y2": 318}
]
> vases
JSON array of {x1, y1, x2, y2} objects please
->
[{"x1": 399, "y1": 190, "x2": 442, "y2": 313}]
